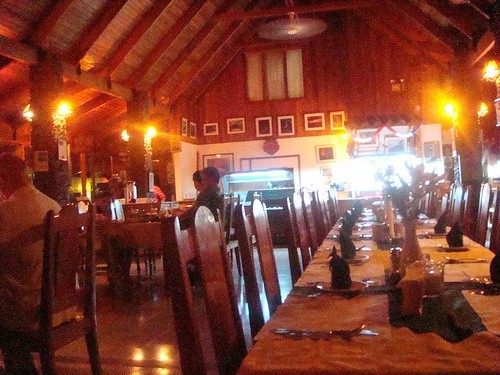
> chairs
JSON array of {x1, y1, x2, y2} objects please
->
[
  {"x1": 420, "y1": 180, "x2": 500, "y2": 255},
  {"x1": 0, "y1": 188, "x2": 340, "y2": 375}
]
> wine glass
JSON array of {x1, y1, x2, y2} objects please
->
[
  {"x1": 150, "y1": 207, "x2": 157, "y2": 222},
  {"x1": 131, "y1": 208, "x2": 139, "y2": 222}
]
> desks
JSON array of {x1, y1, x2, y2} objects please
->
[
  {"x1": 336, "y1": 196, "x2": 378, "y2": 214},
  {"x1": 109, "y1": 223, "x2": 162, "y2": 292},
  {"x1": 239, "y1": 210, "x2": 500, "y2": 375}
]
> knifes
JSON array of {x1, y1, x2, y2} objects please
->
[{"x1": 271, "y1": 328, "x2": 378, "y2": 337}]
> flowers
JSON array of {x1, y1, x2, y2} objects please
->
[{"x1": 374, "y1": 160, "x2": 455, "y2": 221}]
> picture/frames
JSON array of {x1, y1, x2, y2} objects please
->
[
  {"x1": 315, "y1": 145, "x2": 335, "y2": 163},
  {"x1": 255, "y1": 116, "x2": 272, "y2": 137},
  {"x1": 305, "y1": 112, "x2": 325, "y2": 131},
  {"x1": 180, "y1": 117, "x2": 246, "y2": 139},
  {"x1": 278, "y1": 115, "x2": 295, "y2": 136},
  {"x1": 202, "y1": 153, "x2": 234, "y2": 177},
  {"x1": 330, "y1": 110, "x2": 345, "y2": 131}
]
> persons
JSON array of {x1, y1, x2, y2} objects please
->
[
  {"x1": 154, "y1": 165, "x2": 226, "y2": 282},
  {"x1": 0, "y1": 154, "x2": 67, "y2": 375}
]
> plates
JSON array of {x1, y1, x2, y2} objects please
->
[{"x1": 316, "y1": 213, "x2": 494, "y2": 293}]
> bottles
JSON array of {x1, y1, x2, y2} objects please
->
[
  {"x1": 385, "y1": 246, "x2": 403, "y2": 305},
  {"x1": 126, "y1": 180, "x2": 138, "y2": 203}
]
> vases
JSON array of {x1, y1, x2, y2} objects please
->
[{"x1": 402, "y1": 217, "x2": 423, "y2": 263}]
